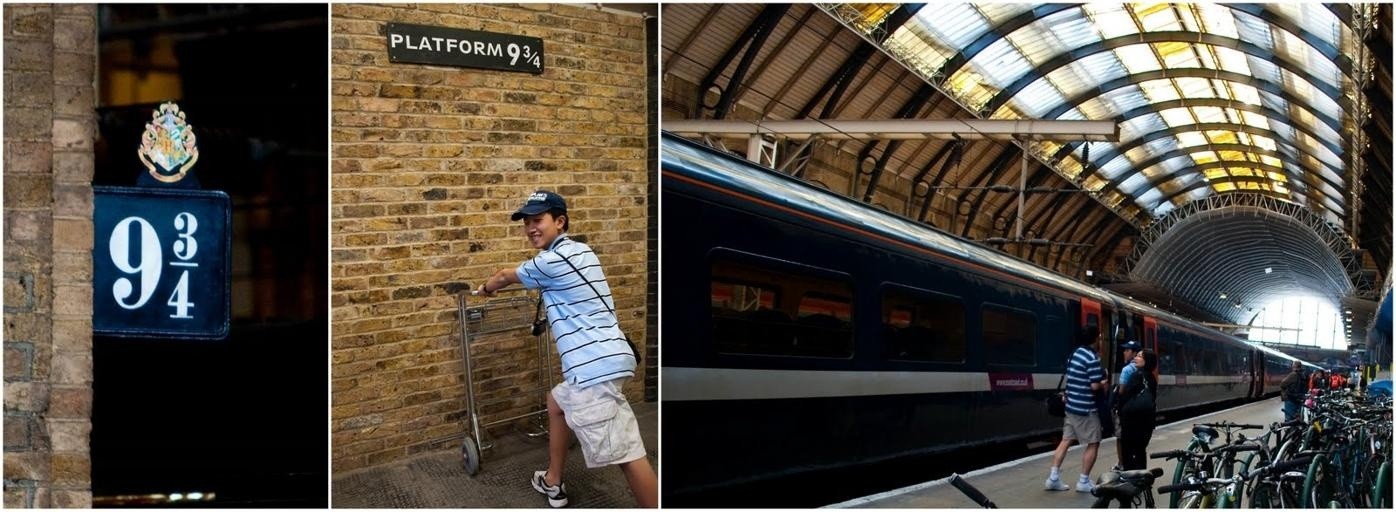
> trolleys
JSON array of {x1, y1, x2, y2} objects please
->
[{"x1": 454, "y1": 284, "x2": 559, "y2": 477}]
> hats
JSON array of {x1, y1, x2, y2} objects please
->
[
  {"x1": 511, "y1": 190, "x2": 566, "y2": 221},
  {"x1": 1119, "y1": 340, "x2": 1141, "y2": 351}
]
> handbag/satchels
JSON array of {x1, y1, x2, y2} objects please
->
[
  {"x1": 1127, "y1": 372, "x2": 1152, "y2": 413},
  {"x1": 1047, "y1": 392, "x2": 1065, "y2": 417}
]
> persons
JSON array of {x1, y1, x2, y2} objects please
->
[
  {"x1": 1115, "y1": 348, "x2": 1158, "y2": 471},
  {"x1": 1111, "y1": 339, "x2": 1143, "y2": 471},
  {"x1": 1043, "y1": 323, "x2": 1111, "y2": 493},
  {"x1": 476, "y1": 189, "x2": 657, "y2": 507},
  {"x1": 1277, "y1": 361, "x2": 1367, "y2": 421}
]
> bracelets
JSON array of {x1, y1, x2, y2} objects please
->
[{"x1": 483, "y1": 283, "x2": 494, "y2": 295}]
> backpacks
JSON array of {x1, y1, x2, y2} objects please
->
[{"x1": 1295, "y1": 370, "x2": 1306, "y2": 394}]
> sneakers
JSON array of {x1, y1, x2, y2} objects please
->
[
  {"x1": 1110, "y1": 464, "x2": 1123, "y2": 473},
  {"x1": 530, "y1": 470, "x2": 568, "y2": 508},
  {"x1": 1075, "y1": 480, "x2": 1095, "y2": 493},
  {"x1": 1044, "y1": 477, "x2": 1069, "y2": 490}
]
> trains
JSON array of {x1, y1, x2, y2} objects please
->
[{"x1": 660, "y1": 138, "x2": 1328, "y2": 507}]
